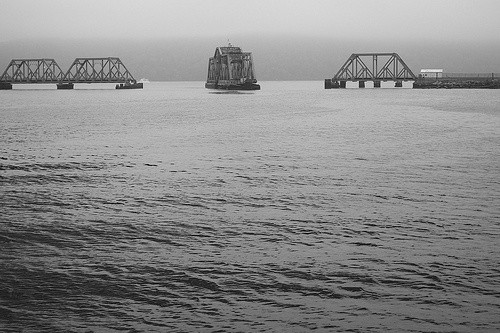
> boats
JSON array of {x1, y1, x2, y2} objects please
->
[
  {"x1": 116, "y1": 78, "x2": 143, "y2": 89},
  {"x1": 331, "y1": 80, "x2": 339, "y2": 88},
  {"x1": 205, "y1": 40, "x2": 261, "y2": 90}
]
